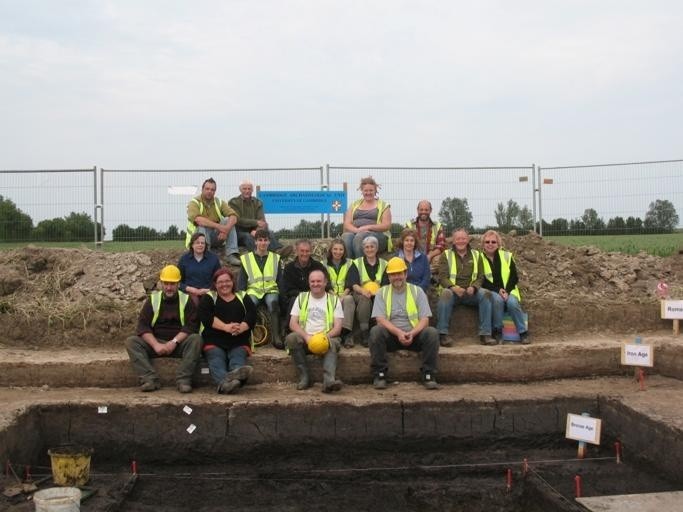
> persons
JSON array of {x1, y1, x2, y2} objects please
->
[{"x1": 125, "y1": 178, "x2": 529, "y2": 393}]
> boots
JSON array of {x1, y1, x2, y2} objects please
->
[
  {"x1": 290, "y1": 346, "x2": 314, "y2": 390},
  {"x1": 269, "y1": 310, "x2": 285, "y2": 350},
  {"x1": 320, "y1": 350, "x2": 344, "y2": 393}
]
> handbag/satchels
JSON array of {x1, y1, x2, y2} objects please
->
[{"x1": 502, "y1": 312, "x2": 529, "y2": 341}]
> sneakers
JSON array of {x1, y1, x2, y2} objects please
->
[
  {"x1": 372, "y1": 365, "x2": 388, "y2": 390},
  {"x1": 361, "y1": 330, "x2": 369, "y2": 347},
  {"x1": 420, "y1": 369, "x2": 438, "y2": 389},
  {"x1": 223, "y1": 365, "x2": 254, "y2": 380},
  {"x1": 519, "y1": 330, "x2": 531, "y2": 344},
  {"x1": 217, "y1": 379, "x2": 240, "y2": 395},
  {"x1": 274, "y1": 244, "x2": 293, "y2": 259},
  {"x1": 439, "y1": 332, "x2": 452, "y2": 347},
  {"x1": 139, "y1": 378, "x2": 162, "y2": 392},
  {"x1": 343, "y1": 330, "x2": 355, "y2": 349},
  {"x1": 177, "y1": 382, "x2": 193, "y2": 393},
  {"x1": 494, "y1": 332, "x2": 504, "y2": 344},
  {"x1": 226, "y1": 251, "x2": 243, "y2": 267},
  {"x1": 479, "y1": 333, "x2": 497, "y2": 346}
]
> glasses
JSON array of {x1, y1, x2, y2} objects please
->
[{"x1": 484, "y1": 240, "x2": 498, "y2": 244}]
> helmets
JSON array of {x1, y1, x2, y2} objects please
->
[
  {"x1": 159, "y1": 264, "x2": 182, "y2": 282},
  {"x1": 361, "y1": 282, "x2": 381, "y2": 296},
  {"x1": 307, "y1": 332, "x2": 330, "y2": 355},
  {"x1": 250, "y1": 325, "x2": 269, "y2": 347},
  {"x1": 385, "y1": 256, "x2": 408, "y2": 274}
]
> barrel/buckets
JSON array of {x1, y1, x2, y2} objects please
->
[
  {"x1": 34, "y1": 487, "x2": 82, "y2": 512},
  {"x1": 47, "y1": 445, "x2": 94, "y2": 486}
]
